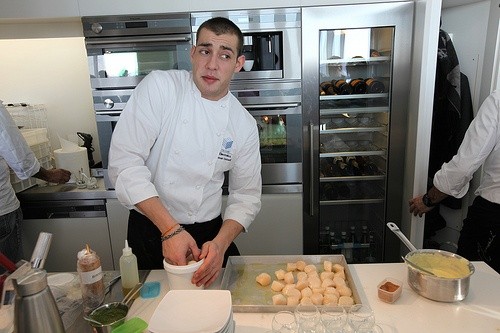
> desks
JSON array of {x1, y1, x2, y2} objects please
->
[{"x1": 123, "y1": 260, "x2": 500, "y2": 333}]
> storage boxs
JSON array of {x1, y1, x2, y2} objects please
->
[{"x1": 4, "y1": 103, "x2": 54, "y2": 193}]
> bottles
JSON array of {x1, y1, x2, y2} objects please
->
[
  {"x1": 320, "y1": 223, "x2": 380, "y2": 264},
  {"x1": 119, "y1": 240, "x2": 139, "y2": 301},
  {"x1": 76, "y1": 245, "x2": 105, "y2": 312},
  {"x1": 320, "y1": 45, "x2": 385, "y2": 95},
  {"x1": 327, "y1": 155, "x2": 372, "y2": 172}
]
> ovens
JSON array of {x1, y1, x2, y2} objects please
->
[{"x1": 81, "y1": 6, "x2": 307, "y2": 189}]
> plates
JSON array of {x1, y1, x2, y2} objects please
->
[{"x1": 147, "y1": 288, "x2": 236, "y2": 333}]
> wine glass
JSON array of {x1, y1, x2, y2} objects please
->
[{"x1": 271, "y1": 301, "x2": 385, "y2": 333}]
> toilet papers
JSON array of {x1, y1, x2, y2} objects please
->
[{"x1": 54, "y1": 135, "x2": 90, "y2": 184}]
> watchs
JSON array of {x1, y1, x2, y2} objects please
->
[{"x1": 422, "y1": 193, "x2": 434, "y2": 207}]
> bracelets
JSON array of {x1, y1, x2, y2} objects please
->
[{"x1": 160, "y1": 223, "x2": 184, "y2": 241}]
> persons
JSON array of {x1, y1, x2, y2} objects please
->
[
  {"x1": 408, "y1": 84, "x2": 500, "y2": 275},
  {"x1": 0, "y1": 100, "x2": 72, "y2": 273},
  {"x1": 108, "y1": 16, "x2": 262, "y2": 288}
]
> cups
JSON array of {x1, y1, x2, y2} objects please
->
[{"x1": 87, "y1": 301, "x2": 129, "y2": 333}]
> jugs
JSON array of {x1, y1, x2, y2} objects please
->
[{"x1": 8, "y1": 257, "x2": 65, "y2": 333}]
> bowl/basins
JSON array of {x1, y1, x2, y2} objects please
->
[
  {"x1": 163, "y1": 253, "x2": 207, "y2": 291},
  {"x1": 385, "y1": 219, "x2": 475, "y2": 303}
]
